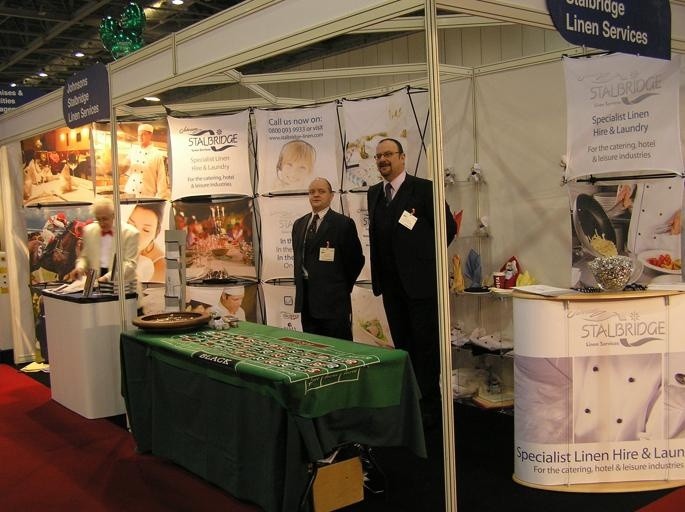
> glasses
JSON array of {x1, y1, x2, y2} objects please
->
[{"x1": 373, "y1": 151, "x2": 401, "y2": 160}]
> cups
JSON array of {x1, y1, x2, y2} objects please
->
[{"x1": 493, "y1": 271, "x2": 506, "y2": 289}]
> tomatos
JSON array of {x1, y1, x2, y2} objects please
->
[{"x1": 649, "y1": 255, "x2": 670, "y2": 266}]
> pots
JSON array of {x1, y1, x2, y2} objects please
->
[{"x1": 571, "y1": 193, "x2": 616, "y2": 253}]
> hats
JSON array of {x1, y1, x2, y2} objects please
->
[
  {"x1": 137, "y1": 124, "x2": 153, "y2": 133},
  {"x1": 222, "y1": 286, "x2": 244, "y2": 296}
]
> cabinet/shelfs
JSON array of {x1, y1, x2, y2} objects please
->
[
  {"x1": 444, "y1": 280, "x2": 515, "y2": 416},
  {"x1": 118, "y1": 320, "x2": 412, "y2": 511}
]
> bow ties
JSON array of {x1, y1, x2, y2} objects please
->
[{"x1": 102, "y1": 230, "x2": 113, "y2": 236}]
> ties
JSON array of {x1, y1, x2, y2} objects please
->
[
  {"x1": 304, "y1": 214, "x2": 318, "y2": 273},
  {"x1": 384, "y1": 183, "x2": 392, "y2": 208}
]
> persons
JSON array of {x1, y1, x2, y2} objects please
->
[
  {"x1": 291, "y1": 177, "x2": 365, "y2": 342},
  {"x1": 366, "y1": 137, "x2": 460, "y2": 458},
  {"x1": 188, "y1": 299, "x2": 213, "y2": 314},
  {"x1": 122, "y1": 124, "x2": 169, "y2": 200},
  {"x1": 615, "y1": 178, "x2": 684, "y2": 257},
  {"x1": 275, "y1": 138, "x2": 318, "y2": 188},
  {"x1": 62, "y1": 196, "x2": 140, "y2": 294},
  {"x1": 126, "y1": 202, "x2": 166, "y2": 282},
  {"x1": 210, "y1": 286, "x2": 246, "y2": 322}
]
